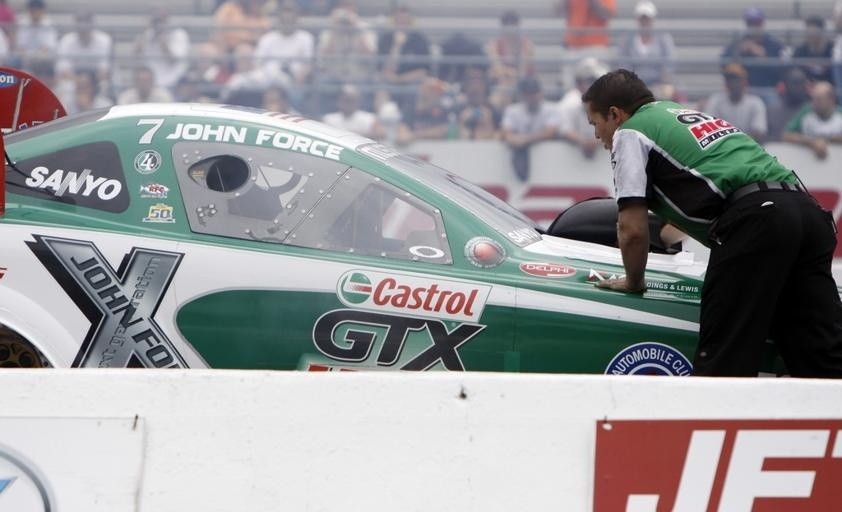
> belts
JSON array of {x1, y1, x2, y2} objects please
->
[{"x1": 724, "y1": 181, "x2": 806, "y2": 211}]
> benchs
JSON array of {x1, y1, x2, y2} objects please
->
[{"x1": 0, "y1": 0, "x2": 841, "y2": 148}]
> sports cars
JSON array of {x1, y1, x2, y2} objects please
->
[{"x1": 1, "y1": 100, "x2": 710, "y2": 374}]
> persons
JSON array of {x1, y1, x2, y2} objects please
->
[
  {"x1": 584, "y1": 70, "x2": 840, "y2": 375},
  {"x1": 1, "y1": 1, "x2": 841, "y2": 164}
]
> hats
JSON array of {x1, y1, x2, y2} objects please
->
[
  {"x1": 744, "y1": 7, "x2": 764, "y2": 23},
  {"x1": 633, "y1": 0, "x2": 657, "y2": 20},
  {"x1": 573, "y1": 57, "x2": 606, "y2": 79}
]
